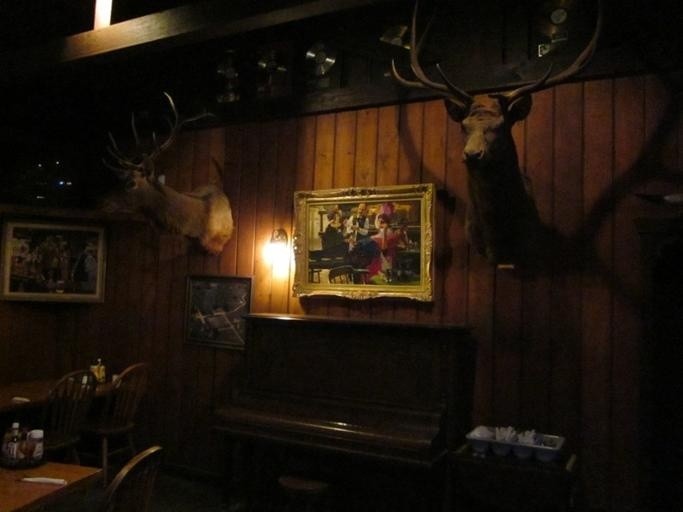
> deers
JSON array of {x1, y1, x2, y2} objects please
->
[
  {"x1": 391, "y1": 0, "x2": 604, "y2": 259},
  {"x1": 100, "y1": 90, "x2": 234, "y2": 255}
]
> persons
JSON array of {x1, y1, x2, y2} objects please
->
[
  {"x1": 324, "y1": 208, "x2": 344, "y2": 246},
  {"x1": 347, "y1": 204, "x2": 370, "y2": 250},
  {"x1": 369, "y1": 214, "x2": 392, "y2": 238}
]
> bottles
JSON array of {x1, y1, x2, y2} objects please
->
[{"x1": 2, "y1": 422, "x2": 44, "y2": 460}]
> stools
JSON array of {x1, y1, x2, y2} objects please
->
[{"x1": 278, "y1": 474, "x2": 329, "y2": 512}]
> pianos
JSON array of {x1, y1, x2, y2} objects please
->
[{"x1": 200, "y1": 311, "x2": 476, "y2": 510}]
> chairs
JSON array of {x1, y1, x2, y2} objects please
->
[
  {"x1": 94, "y1": 444, "x2": 165, "y2": 511},
  {"x1": 37, "y1": 363, "x2": 151, "y2": 488}
]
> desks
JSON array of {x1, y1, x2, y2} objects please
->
[
  {"x1": 2, "y1": 457, "x2": 101, "y2": 511},
  {"x1": 0, "y1": 377, "x2": 149, "y2": 412}
]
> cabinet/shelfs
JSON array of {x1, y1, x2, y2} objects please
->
[{"x1": 446, "y1": 443, "x2": 581, "y2": 512}]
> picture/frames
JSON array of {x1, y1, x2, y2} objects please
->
[
  {"x1": 292, "y1": 181, "x2": 438, "y2": 304},
  {"x1": 182, "y1": 274, "x2": 252, "y2": 352},
  {"x1": 2, "y1": 222, "x2": 106, "y2": 304}
]
